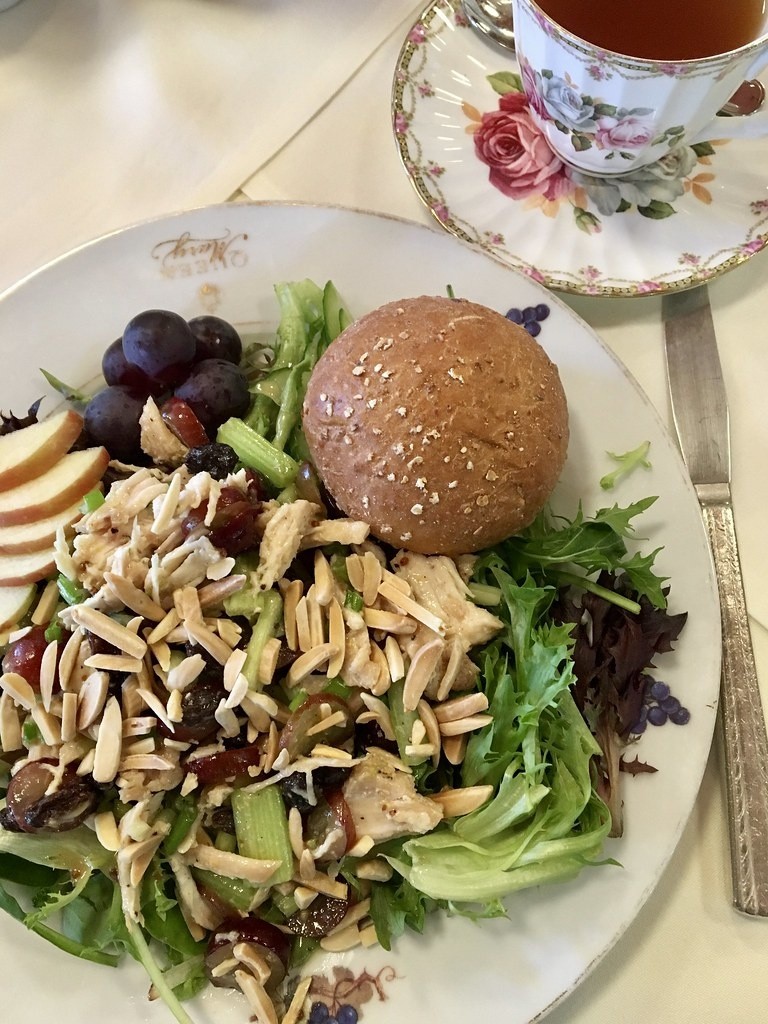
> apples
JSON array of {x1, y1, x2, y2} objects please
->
[{"x1": 0, "y1": 413, "x2": 108, "y2": 631}]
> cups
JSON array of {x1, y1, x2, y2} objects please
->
[{"x1": 512, "y1": 0, "x2": 768, "y2": 178}]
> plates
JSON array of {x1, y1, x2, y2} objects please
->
[
  {"x1": 0, "y1": 203, "x2": 724, "y2": 1023},
  {"x1": 391, "y1": 0, "x2": 767, "y2": 299}
]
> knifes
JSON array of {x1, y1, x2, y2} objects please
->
[{"x1": 663, "y1": 285, "x2": 768, "y2": 919}]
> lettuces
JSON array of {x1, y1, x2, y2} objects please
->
[{"x1": 1, "y1": 282, "x2": 673, "y2": 1023}]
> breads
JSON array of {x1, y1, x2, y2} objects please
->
[{"x1": 298, "y1": 297, "x2": 569, "y2": 554}]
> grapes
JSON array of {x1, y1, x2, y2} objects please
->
[{"x1": 86, "y1": 308, "x2": 248, "y2": 454}]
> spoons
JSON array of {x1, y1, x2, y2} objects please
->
[{"x1": 463, "y1": 1, "x2": 764, "y2": 116}]
